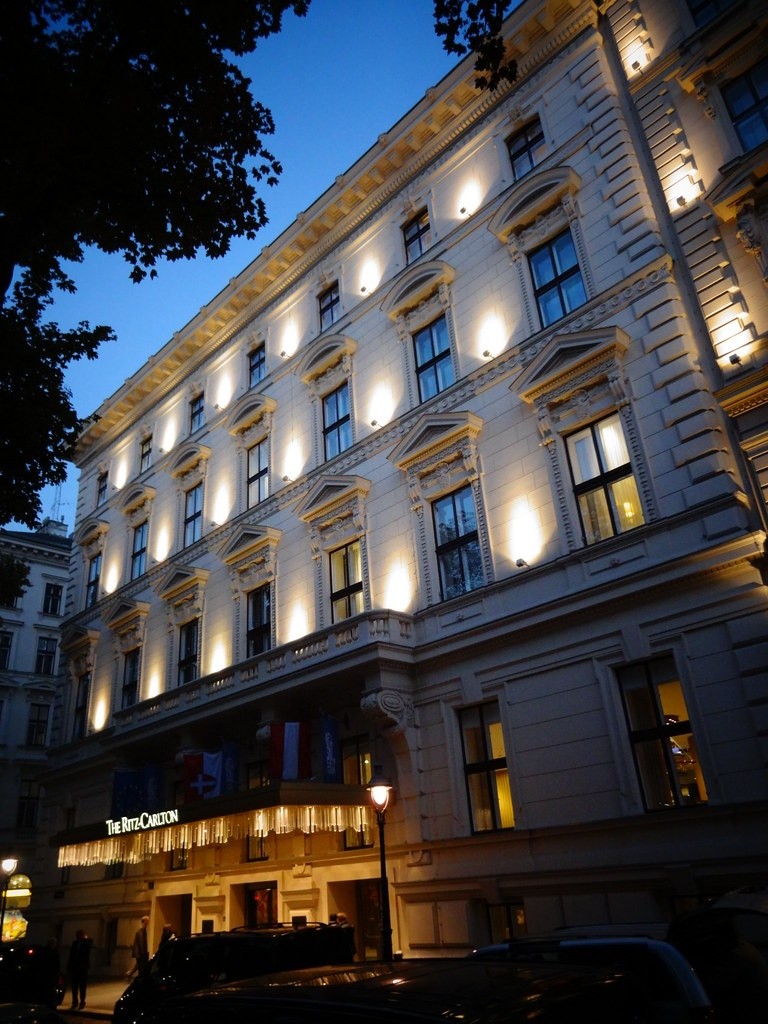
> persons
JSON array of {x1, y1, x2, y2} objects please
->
[
  {"x1": 43, "y1": 937, "x2": 61, "y2": 1011},
  {"x1": 66, "y1": 929, "x2": 93, "y2": 1010},
  {"x1": 159, "y1": 923, "x2": 176, "y2": 949},
  {"x1": 328, "y1": 912, "x2": 353, "y2": 928},
  {"x1": 126, "y1": 917, "x2": 149, "y2": 977}
]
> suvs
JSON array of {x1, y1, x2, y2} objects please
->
[
  {"x1": 111, "y1": 922, "x2": 353, "y2": 1024},
  {"x1": 463, "y1": 920, "x2": 768, "y2": 1024},
  {"x1": 155, "y1": 958, "x2": 688, "y2": 1024},
  {"x1": 713, "y1": 886, "x2": 768, "y2": 913}
]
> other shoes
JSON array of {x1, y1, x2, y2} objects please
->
[
  {"x1": 71, "y1": 1001, "x2": 78, "y2": 1009},
  {"x1": 79, "y1": 1001, "x2": 85, "y2": 1009}
]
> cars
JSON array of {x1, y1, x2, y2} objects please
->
[{"x1": 0, "y1": 1003, "x2": 67, "y2": 1024}]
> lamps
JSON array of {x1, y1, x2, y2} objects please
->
[
  {"x1": 727, "y1": 355, "x2": 743, "y2": 371},
  {"x1": 515, "y1": 558, "x2": 530, "y2": 569},
  {"x1": 677, "y1": 196, "x2": 688, "y2": 207},
  {"x1": 632, "y1": 60, "x2": 644, "y2": 76}
]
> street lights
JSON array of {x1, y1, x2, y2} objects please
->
[
  {"x1": 0, "y1": 857, "x2": 19, "y2": 935},
  {"x1": 365, "y1": 764, "x2": 394, "y2": 961}
]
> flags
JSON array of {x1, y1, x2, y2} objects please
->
[
  {"x1": 266, "y1": 721, "x2": 311, "y2": 781},
  {"x1": 321, "y1": 716, "x2": 340, "y2": 784},
  {"x1": 111, "y1": 763, "x2": 166, "y2": 821},
  {"x1": 182, "y1": 743, "x2": 240, "y2": 805}
]
